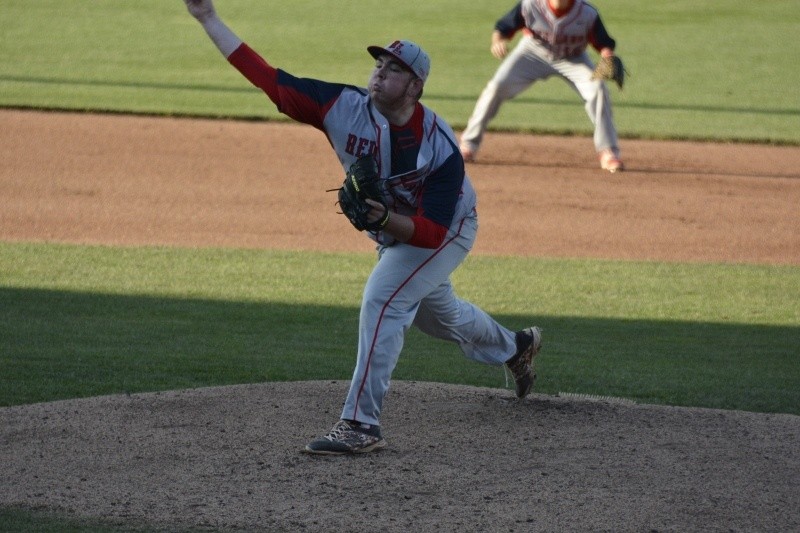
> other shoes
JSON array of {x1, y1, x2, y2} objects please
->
[
  {"x1": 459, "y1": 139, "x2": 475, "y2": 163},
  {"x1": 600, "y1": 150, "x2": 623, "y2": 171}
]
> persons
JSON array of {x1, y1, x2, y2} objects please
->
[
  {"x1": 458, "y1": 0, "x2": 626, "y2": 171},
  {"x1": 177, "y1": 1, "x2": 544, "y2": 456}
]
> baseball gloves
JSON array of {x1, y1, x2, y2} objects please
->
[
  {"x1": 338, "y1": 153, "x2": 390, "y2": 232},
  {"x1": 591, "y1": 56, "x2": 623, "y2": 91}
]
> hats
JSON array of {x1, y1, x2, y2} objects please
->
[{"x1": 367, "y1": 40, "x2": 431, "y2": 85}]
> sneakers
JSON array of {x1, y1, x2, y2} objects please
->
[
  {"x1": 305, "y1": 419, "x2": 386, "y2": 454},
  {"x1": 501, "y1": 326, "x2": 543, "y2": 400}
]
